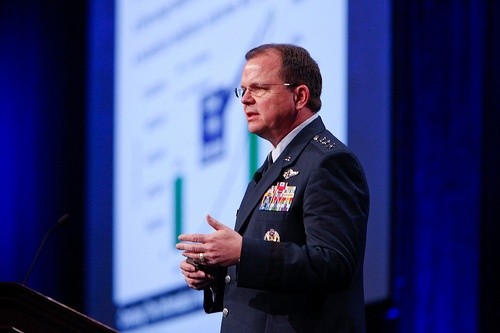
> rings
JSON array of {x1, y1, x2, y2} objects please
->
[{"x1": 199, "y1": 252, "x2": 204, "y2": 262}]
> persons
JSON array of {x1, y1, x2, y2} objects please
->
[{"x1": 176, "y1": 43, "x2": 369, "y2": 333}]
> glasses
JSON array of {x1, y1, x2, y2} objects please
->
[{"x1": 234, "y1": 83, "x2": 295, "y2": 99}]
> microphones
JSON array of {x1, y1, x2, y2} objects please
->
[
  {"x1": 254, "y1": 172, "x2": 262, "y2": 184},
  {"x1": 22, "y1": 213, "x2": 69, "y2": 285}
]
